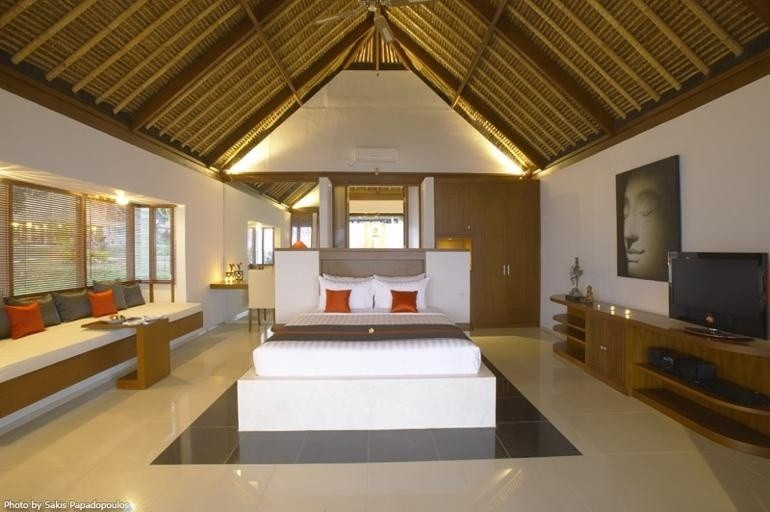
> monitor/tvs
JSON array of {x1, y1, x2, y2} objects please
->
[{"x1": 668, "y1": 252, "x2": 770, "y2": 342}]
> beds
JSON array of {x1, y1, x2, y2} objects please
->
[{"x1": 235, "y1": 247, "x2": 498, "y2": 432}]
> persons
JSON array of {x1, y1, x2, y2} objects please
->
[{"x1": 621, "y1": 168, "x2": 678, "y2": 278}]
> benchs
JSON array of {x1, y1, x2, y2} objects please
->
[{"x1": 1, "y1": 302, "x2": 205, "y2": 418}]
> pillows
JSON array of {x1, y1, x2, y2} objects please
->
[
  {"x1": 311, "y1": 267, "x2": 431, "y2": 316},
  {"x1": 2, "y1": 278, "x2": 147, "y2": 340}
]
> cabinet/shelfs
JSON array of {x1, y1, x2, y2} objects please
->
[
  {"x1": 546, "y1": 293, "x2": 770, "y2": 462},
  {"x1": 433, "y1": 175, "x2": 541, "y2": 329}
]
